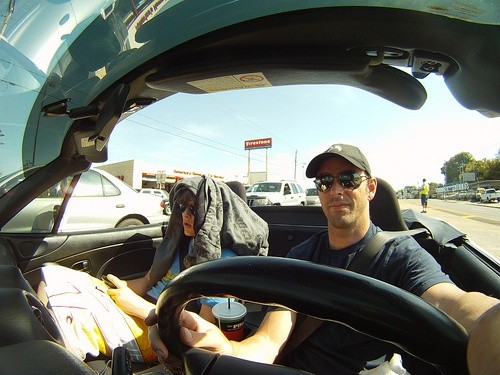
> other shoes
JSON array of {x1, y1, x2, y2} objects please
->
[{"x1": 421, "y1": 211, "x2": 427, "y2": 213}]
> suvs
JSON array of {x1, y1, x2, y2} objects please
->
[{"x1": 246, "y1": 179, "x2": 307, "y2": 208}]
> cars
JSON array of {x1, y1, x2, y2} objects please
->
[
  {"x1": 137, "y1": 187, "x2": 172, "y2": 216},
  {"x1": 2, "y1": 163, "x2": 165, "y2": 231},
  {"x1": 305, "y1": 187, "x2": 322, "y2": 206},
  {"x1": 442, "y1": 188, "x2": 485, "y2": 200}
]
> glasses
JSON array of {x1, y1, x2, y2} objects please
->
[
  {"x1": 313, "y1": 171, "x2": 369, "y2": 192},
  {"x1": 178, "y1": 202, "x2": 196, "y2": 216}
]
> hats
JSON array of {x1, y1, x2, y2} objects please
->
[{"x1": 306, "y1": 143, "x2": 371, "y2": 180}]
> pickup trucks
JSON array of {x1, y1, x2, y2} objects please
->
[{"x1": 481, "y1": 188, "x2": 500, "y2": 202}]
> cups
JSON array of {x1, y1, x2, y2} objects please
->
[{"x1": 211, "y1": 297, "x2": 248, "y2": 342}]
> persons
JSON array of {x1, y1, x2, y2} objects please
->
[
  {"x1": 37, "y1": 176, "x2": 269, "y2": 364},
  {"x1": 143, "y1": 144, "x2": 500, "y2": 375},
  {"x1": 417, "y1": 179, "x2": 429, "y2": 213}
]
